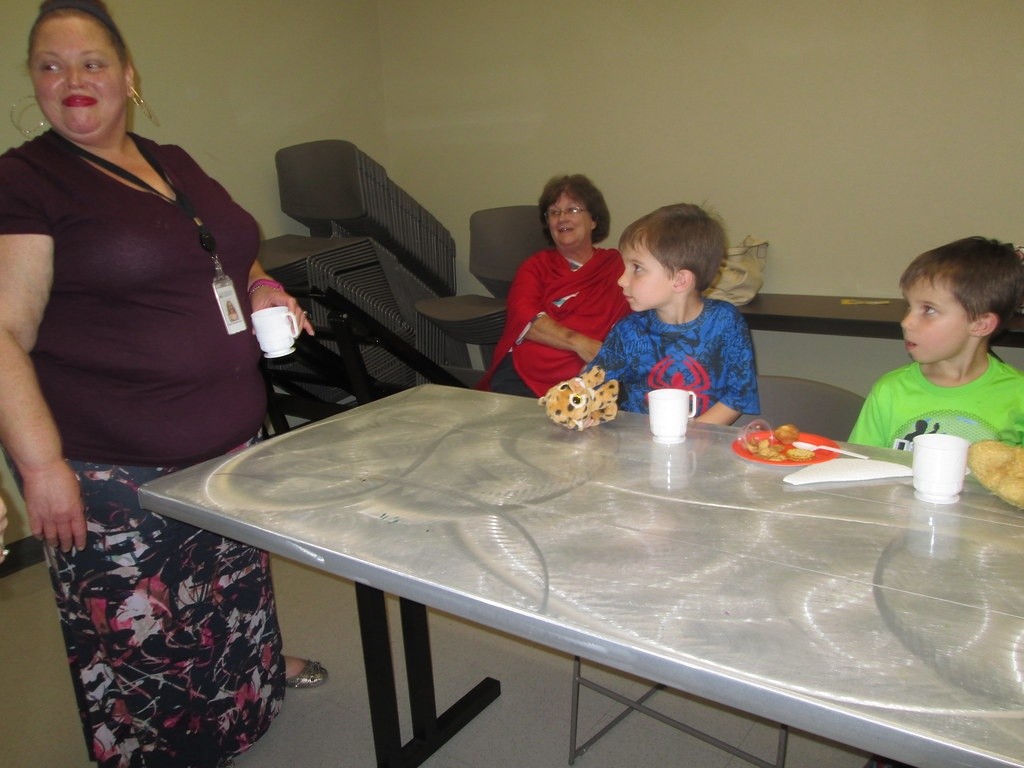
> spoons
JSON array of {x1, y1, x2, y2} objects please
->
[{"x1": 792, "y1": 441, "x2": 870, "y2": 460}]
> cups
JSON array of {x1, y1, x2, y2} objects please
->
[
  {"x1": 912, "y1": 434, "x2": 969, "y2": 504},
  {"x1": 648, "y1": 389, "x2": 696, "y2": 443},
  {"x1": 739, "y1": 419, "x2": 773, "y2": 448},
  {"x1": 250, "y1": 306, "x2": 299, "y2": 358}
]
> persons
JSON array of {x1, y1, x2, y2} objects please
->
[
  {"x1": 0, "y1": 0, "x2": 330, "y2": 768},
  {"x1": 842, "y1": 235, "x2": 1024, "y2": 452},
  {"x1": 472, "y1": 175, "x2": 632, "y2": 400},
  {"x1": 226, "y1": 301, "x2": 239, "y2": 322},
  {"x1": 581, "y1": 203, "x2": 760, "y2": 426}
]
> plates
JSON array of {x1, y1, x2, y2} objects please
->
[{"x1": 733, "y1": 432, "x2": 841, "y2": 466}]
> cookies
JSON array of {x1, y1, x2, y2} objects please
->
[{"x1": 748, "y1": 424, "x2": 815, "y2": 461}]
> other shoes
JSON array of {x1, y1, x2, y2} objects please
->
[{"x1": 283, "y1": 656, "x2": 328, "y2": 689}]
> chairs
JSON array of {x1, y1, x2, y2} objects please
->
[
  {"x1": 255, "y1": 141, "x2": 552, "y2": 438},
  {"x1": 567, "y1": 371, "x2": 866, "y2": 763}
]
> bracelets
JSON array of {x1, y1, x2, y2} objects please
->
[{"x1": 248, "y1": 279, "x2": 284, "y2": 298}]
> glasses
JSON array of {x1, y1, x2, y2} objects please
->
[{"x1": 544, "y1": 206, "x2": 585, "y2": 218}]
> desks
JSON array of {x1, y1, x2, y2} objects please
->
[
  {"x1": 138, "y1": 380, "x2": 1023, "y2": 767},
  {"x1": 739, "y1": 293, "x2": 1023, "y2": 348}
]
